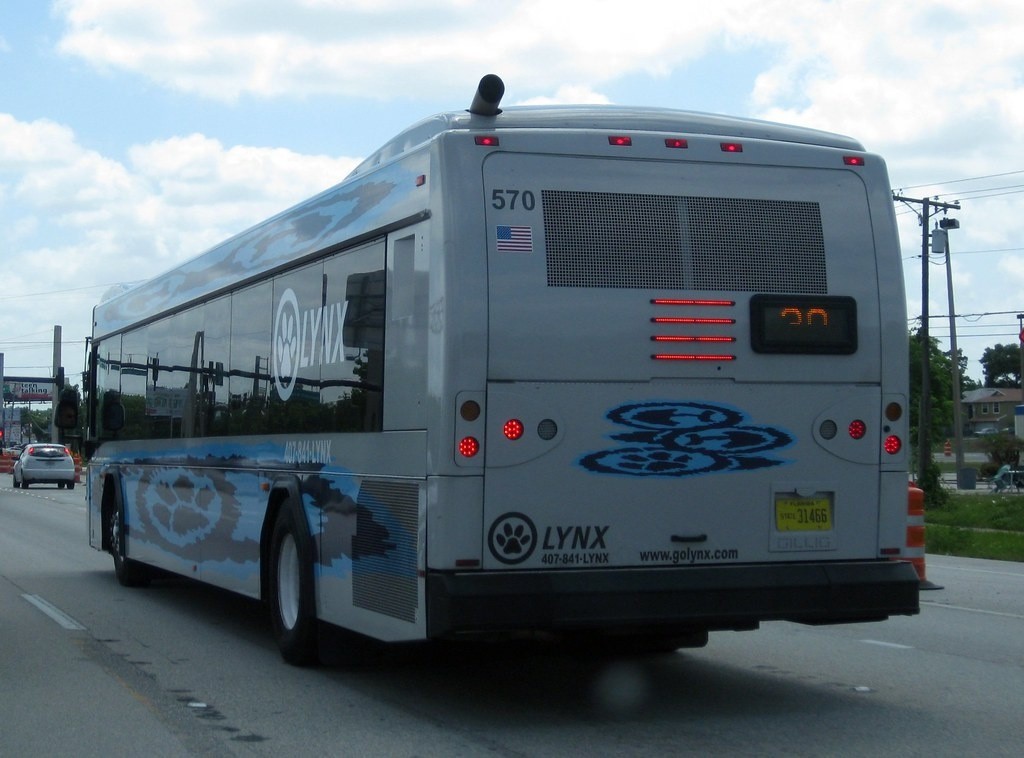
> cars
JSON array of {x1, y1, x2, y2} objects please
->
[{"x1": 12, "y1": 443, "x2": 76, "y2": 490}]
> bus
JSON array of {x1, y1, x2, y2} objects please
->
[{"x1": 52, "y1": 74, "x2": 923, "y2": 667}]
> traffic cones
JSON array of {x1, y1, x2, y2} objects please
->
[{"x1": 74, "y1": 458, "x2": 82, "y2": 483}]
